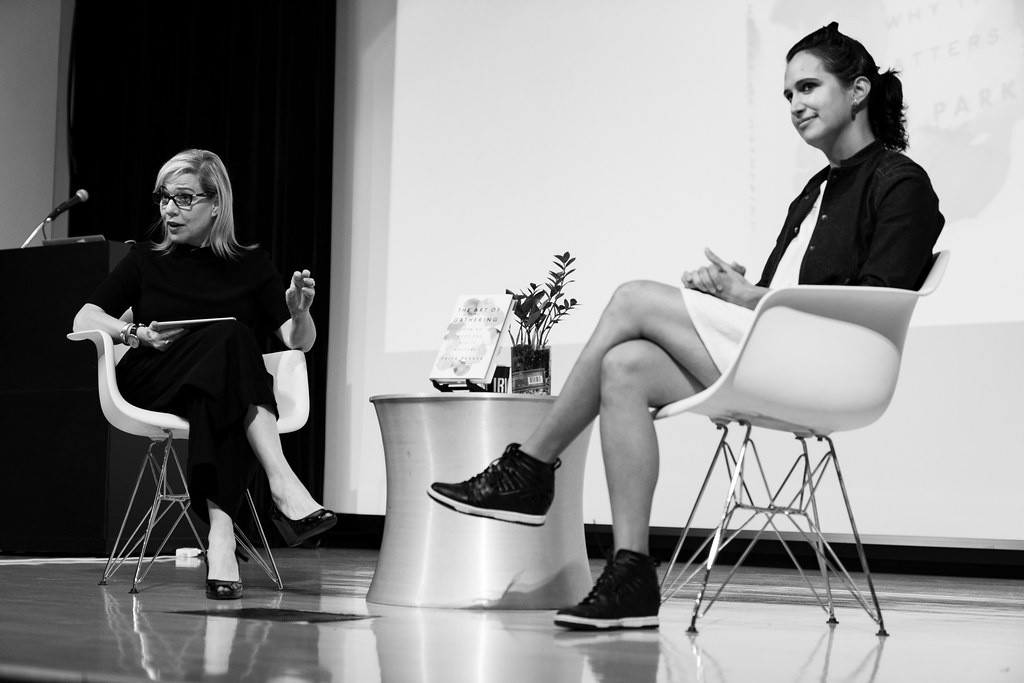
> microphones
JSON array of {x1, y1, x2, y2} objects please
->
[{"x1": 49, "y1": 190, "x2": 88, "y2": 221}]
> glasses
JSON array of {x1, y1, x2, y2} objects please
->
[{"x1": 152, "y1": 189, "x2": 216, "y2": 211}]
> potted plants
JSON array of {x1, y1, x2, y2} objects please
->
[{"x1": 508, "y1": 248, "x2": 583, "y2": 394}]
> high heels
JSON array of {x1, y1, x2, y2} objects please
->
[
  {"x1": 202, "y1": 550, "x2": 244, "y2": 599},
  {"x1": 270, "y1": 501, "x2": 336, "y2": 548}
]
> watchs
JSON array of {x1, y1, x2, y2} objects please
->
[{"x1": 128, "y1": 323, "x2": 145, "y2": 349}]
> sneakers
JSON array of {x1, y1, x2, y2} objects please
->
[
  {"x1": 553, "y1": 548, "x2": 661, "y2": 630},
  {"x1": 427, "y1": 443, "x2": 562, "y2": 525}
]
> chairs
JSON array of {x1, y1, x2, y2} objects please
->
[
  {"x1": 68, "y1": 306, "x2": 313, "y2": 593},
  {"x1": 651, "y1": 249, "x2": 951, "y2": 638}
]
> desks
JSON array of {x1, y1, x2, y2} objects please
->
[{"x1": 367, "y1": 391, "x2": 596, "y2": 611}]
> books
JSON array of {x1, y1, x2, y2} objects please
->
[{"x1": 429, "y1": 293, "x2": 518, "y2": 384}]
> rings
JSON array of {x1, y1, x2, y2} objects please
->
[{"x1": 310, "y1": 278, "x2": 315, "y2": 288}]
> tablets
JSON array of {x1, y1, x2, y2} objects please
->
[{"x1": 157, "y1": 317, "x2": 237, "y2": 331}]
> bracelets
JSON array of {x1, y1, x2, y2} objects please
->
[{"x1": 120, "y1": 323, "x2": 135, "y2": 346}]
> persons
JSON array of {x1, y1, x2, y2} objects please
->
[
  {"x1": 426, "y1": 21, "x2": 945, "y2": 632},
  {"x1": 73, "y1": 149, "x2": 337, "y2": 600}
]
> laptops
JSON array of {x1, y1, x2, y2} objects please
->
[{"x1": 41, "y1": 235, "x2": 105, "y2": 245}]
